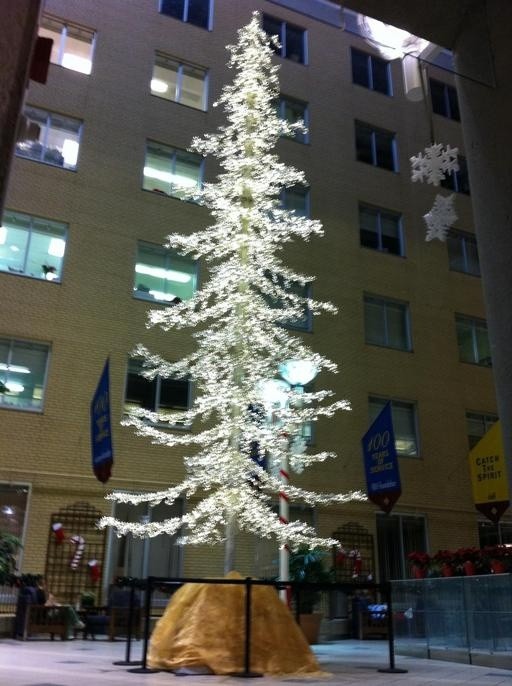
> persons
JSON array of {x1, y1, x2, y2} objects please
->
[{"x1": 28, "y1": 574, "x2": 86, "y2": 641}]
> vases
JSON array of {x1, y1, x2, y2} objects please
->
[{"x1": 412, "y1": 560, "x2": 506, "y2": 579}]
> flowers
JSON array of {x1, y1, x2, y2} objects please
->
[{"x1": 407, "y1": 544, "x2": 512, "y2": 566}]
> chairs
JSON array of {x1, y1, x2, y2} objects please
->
[
  {"x1": 13, "y1": 577, "x2": 144, "y2": 643},
  {"x1": 352, "y1": 590, "x2": 402, "y2": 641}
]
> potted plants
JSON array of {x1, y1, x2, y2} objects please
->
[{"x1": 286, "y1": 544, "x2": 326, "y2": 645}]
